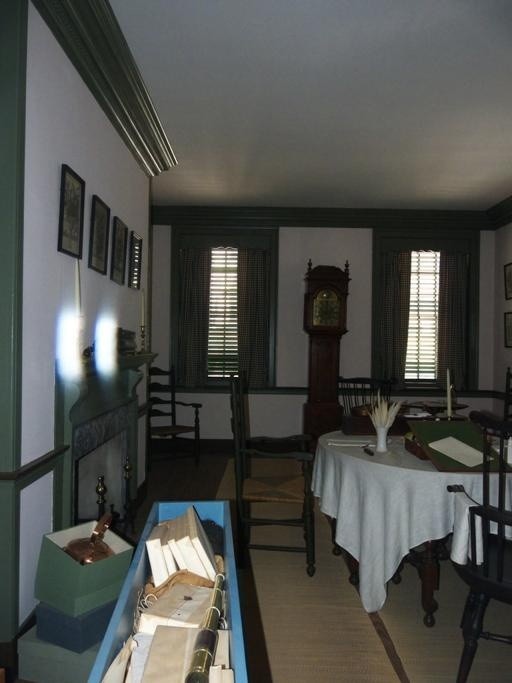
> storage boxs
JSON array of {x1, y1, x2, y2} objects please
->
[
  {"x1": 30, "y1": 571, "x2": 127, "y2": 617},
  {"x1": 85, "y1": 496, "x2": 249, "y2": 683},
  {"x1": 33, "y1": 518, "x2": 136, "y2": 592},
  {"x1": 35, "y1": 599, "x2": 121, "y2": 654}
]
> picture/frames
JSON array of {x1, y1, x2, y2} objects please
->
[
  {"x1": 87, "y1": 194, "x2": 113, "y2": 275},
  {"x1": 503, "y1": 262, "x2": 511, "y2": 301},
  {"x1": 110, "y1": 215, "x2": 129, "y2": 286},
  {"x1": 57, "y1": 162, "x2": 85, "y2": 260},
  {"x1": 128, "y1": 229, "x2": 145, "y2": 290},
  {"x1": 504, "y1": 311, "x2": 512, "y2": 348}
]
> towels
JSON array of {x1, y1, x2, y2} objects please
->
[{"x1": 450, "y1": 490, "x2": 484, "y2": 570}]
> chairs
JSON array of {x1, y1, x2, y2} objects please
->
[
  {"x1": 337, "y1": 376, "x2": 399, "y2": 419},
  {"x1": 228, "y1": 368, "x2": 318, "y2": 578},
  {"x1": 468, "y1": 366, "x2": 512, "y2": 432},
  {"x1": 445, "y1": 409, "x2": 511, "y2": 681},
  {"x1": 146, "y1": 365, "x2": 206, "y2": 468}
]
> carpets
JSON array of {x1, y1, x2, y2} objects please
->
[{"x1": 212, "y1": 448, "x2": 512, "y2": 683}]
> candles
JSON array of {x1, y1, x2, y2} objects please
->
[{"x1": 445, "y1": 368, "x2": 452, "y2": 416}]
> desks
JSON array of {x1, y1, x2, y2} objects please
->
[{"x1": 402, "y1": 400, "x2": 470, "y2": 420}]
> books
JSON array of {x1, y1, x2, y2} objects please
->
[{"x1": 125, "y1": 506, "x2": 235, "y2": 683}]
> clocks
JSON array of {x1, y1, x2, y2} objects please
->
[{"x1": 299, "y1": 255, "x2": 352, "y2": 452}]
